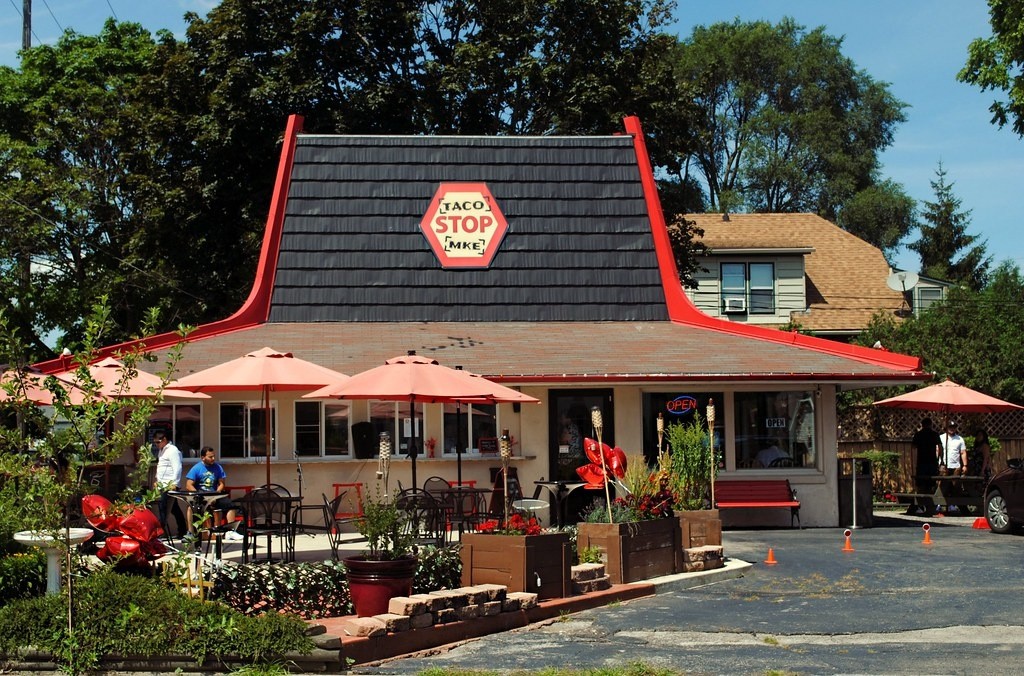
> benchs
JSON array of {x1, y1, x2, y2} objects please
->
[
  {"x1": 714, "y1": 479, "x2": 802, "y2": 530},
  {"x1": 893, "y1": 493, "x2": 935, "y2": 505}
]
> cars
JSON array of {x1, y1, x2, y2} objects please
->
[{"x1": 984, "y1": 456, "x2": 1024, "y2": 533}]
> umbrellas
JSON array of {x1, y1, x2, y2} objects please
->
[
  {"x1": 0, "y1": 356, "x2": 214, "y2": 497},
  {"x1": 301, "y1": 350, "x2": 541, "y2": 553},
  {"x1": 152, "y1": 346, "x2": 352, "y2": 559},
  {"x1": 872, "y1": 375, "x2": 1024, "y2": 476}
]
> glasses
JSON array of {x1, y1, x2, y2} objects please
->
[{"x1": 154, "y1": 439, "x2": 163, "y2": 443}]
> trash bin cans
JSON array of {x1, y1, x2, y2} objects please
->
[{"x1": 837, "y1": 457, "x2": 874, "y2": 528}]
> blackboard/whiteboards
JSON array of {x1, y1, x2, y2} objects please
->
[
  {"x1": 478, "y1": 438, "x2": 499, "y2": 454},
  {"x1": 490, "y1": 466, "x2": 523, "y2": 510}
]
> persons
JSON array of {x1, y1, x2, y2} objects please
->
[
  {"x1": 151, "y1": 430, "x2": 187, "y2": 540},
  {"x1": 182, "y1": 446, "x2": 243, "y2": 542},
  {"x1": 972, "y1": 430, "x2": 992, "y2": 498},
  {"x1": 756, "y1": 438, "x2": 790, "y2": 468},
  {"x1": 911, "y1": 417, "x2": 943, "y2": 514},
  {"x1": 553, "y1": 409, "x2": 586, "y2": 478},
  {"x1": 936, "y1": 420, "x2": 967, "y2": 499}
]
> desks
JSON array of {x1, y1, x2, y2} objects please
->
[
  {"x1": 425, "y1": 487, "x2": 492, "y2": 540},
  {"x1": 167, "y1": 491, "x2": 231, "y2": 555},
  {"x1": 534, "y1": 480, "x2": 588, "y2": 532},
  {"x1": 930, "y1": 474, "x2": 986, "y2": 506},
  {"x1": 232, "y1": 497, "x2": 305, "y2": 565},
  {"x1": 381, "y1": 504, "x2": 455, "y2": 554}
]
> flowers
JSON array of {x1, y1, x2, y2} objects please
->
[
  {"x1": 472, "y1": 506, "x2": 547, "y2": 536},
  {"x1": 587, "y1": 491, "x2": 674, "y2": 526}
]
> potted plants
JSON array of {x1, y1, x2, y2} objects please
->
[
  {"x1": 656, "y1": 409, "x2": 723, "y2": 549},
  {"x1": 336, "y1": 480, "x2": 417, "y2": 618}
]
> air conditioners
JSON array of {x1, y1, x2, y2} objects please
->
[{"x1": 723, "y1": 298, "x2": 746, "y2": 312}]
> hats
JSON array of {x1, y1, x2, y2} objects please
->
[{"x1": 948, "y1": 420, "x2": 958, "y2": 428}]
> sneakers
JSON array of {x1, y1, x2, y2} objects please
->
[
  {"x1": 181, "y1": 532, "x2": 194, "y2": 543},
  {"x1": 225, "y1": 530, "x2": 244, "y2": 541}
]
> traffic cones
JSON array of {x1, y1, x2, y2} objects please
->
[{"x1": 764, "y1": 548, "x2": 777, "y2": 564}]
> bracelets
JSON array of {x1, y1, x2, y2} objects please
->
[{"x1": 963, "y1": 465, "x2": 967, "y2": 467}]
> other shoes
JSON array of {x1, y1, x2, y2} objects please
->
[
  {"x1": 936, "y1": 505, "x2": 942, "y2": 511},
  {"x1": 162, "y1": 534, "x2": 177, "y2": 541},
  {"x1": 916, "y1": 496, "x2": 924, "y2": 512},
  {"x1": 950, "y1": 505, "x2": 957, "y2": 511}
]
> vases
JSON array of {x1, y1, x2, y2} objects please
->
[
  {"x1": 577, "y1": 517, "x2": 682, "y2": 584},
  {"x1": 461, "y1": 533, "x2": 573, "y2": 598}
]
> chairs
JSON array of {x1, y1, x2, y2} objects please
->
[{"x1": 136, "y1": 481, "x2": 562, "y2": 565}]
